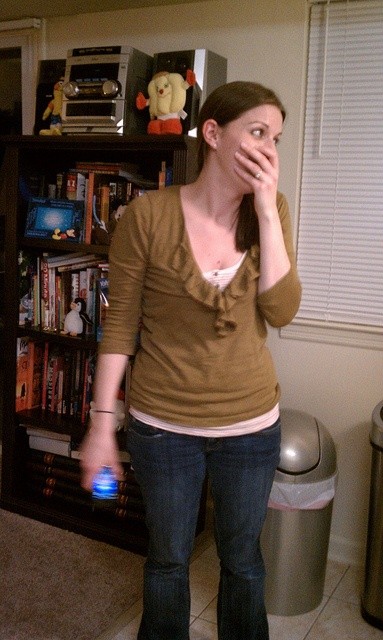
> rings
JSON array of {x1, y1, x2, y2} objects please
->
[{"x1": 256, "y1": 166, "x2": 263, "y2": 182}]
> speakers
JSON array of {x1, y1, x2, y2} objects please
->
[
  {"x1": 152, "y1": 49, "x2": 228, "y2": 135},
  {"x1": 33, "y1": 57, "x2": 66, "y2": 134}
]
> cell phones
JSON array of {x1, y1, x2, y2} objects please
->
[{"x1": 90, "y1": 464, "x2": 120, "y2": 502}]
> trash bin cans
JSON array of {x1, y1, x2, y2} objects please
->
[
  {"x1": 261, "y1": 408, "x2": 337, "y2": 617},
  {"x1": 360, "y1": 399, "x2": 383, "y2": 633}
]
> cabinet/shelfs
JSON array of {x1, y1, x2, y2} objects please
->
[{"x1": 0, "y1": 134, "x2": 205, "y2": 560}]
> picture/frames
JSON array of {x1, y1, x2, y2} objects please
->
[{"x1": 23, "y1": 194, "x2": 83, "y2": 243}]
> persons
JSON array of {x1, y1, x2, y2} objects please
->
[{"x1": 79, "y1": 81, "x2": 303, "y2": 640}]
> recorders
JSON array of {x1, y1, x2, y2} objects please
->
[{"x1": 60, "y1": 45, "x2": 153, "y2": 135}]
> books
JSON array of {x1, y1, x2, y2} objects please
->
[
  {"x1": 17, "y1": 247, "x2": 112, "y2": 344},
  {"x1": 13, "y1": 333, "x2": 131, "y2": 430},
  {"x1": 8, "y1": 423, "x2": 142, "y2": 530},
  {"x1": 19, "y1": 157, "x2": 174, "y2": 247}
]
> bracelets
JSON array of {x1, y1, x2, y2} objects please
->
[{"x1": 89, "y1": 407, "x2": 119, "y2": 416}]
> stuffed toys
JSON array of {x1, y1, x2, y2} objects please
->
[
  {"x1": 137, "y1": 66, "x2": 195, "y2": 135},
  {"x1": 39, "y1": 78, "x2": 64, "y2": 137}
]
